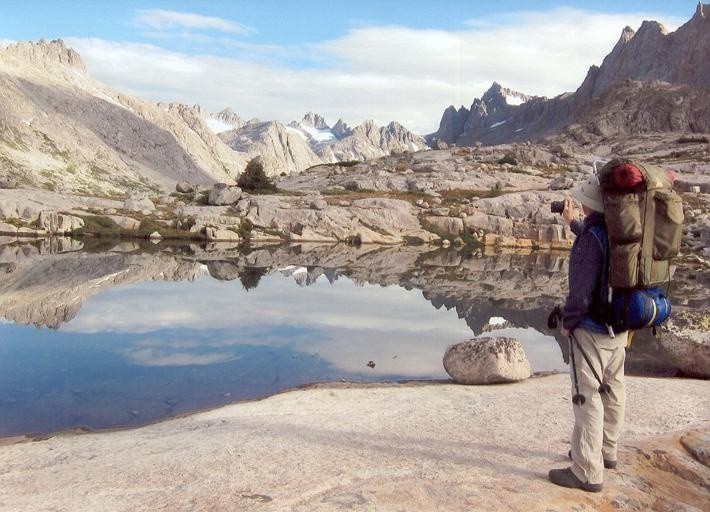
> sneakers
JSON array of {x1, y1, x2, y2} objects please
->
[
  {"x1": 549, "y1": 467, "x2": 603, "y2": 491},
  {"x1": 569, "y1": 449, "x2": 617, "y2": 468}
]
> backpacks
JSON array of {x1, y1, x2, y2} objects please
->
[{"x1": 597, "y1": 157, "x2": 684, "y2": 329}]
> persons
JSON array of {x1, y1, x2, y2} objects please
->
[{"x1": 549, "y1": 174, "x2": 627, "y2": 492}]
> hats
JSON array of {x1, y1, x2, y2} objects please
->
[{"x1": 571, "y1": 176, "x2": 605, "y2": 214}]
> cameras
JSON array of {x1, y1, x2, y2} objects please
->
[{"x1": 550, "y1": 200, "x2": 564, "y2": 213}]
